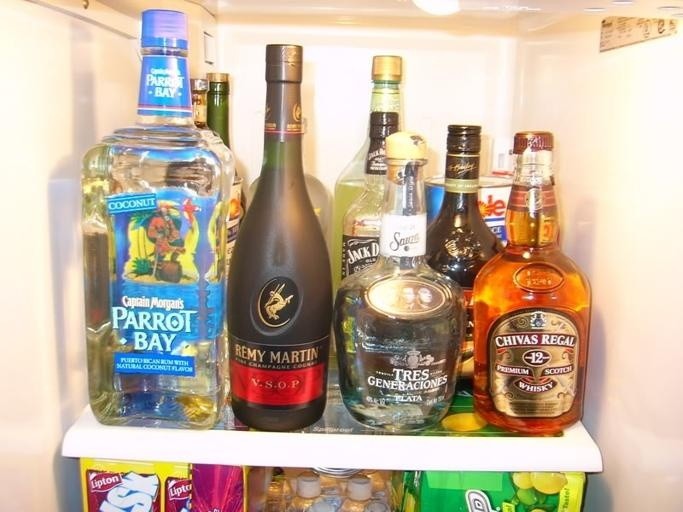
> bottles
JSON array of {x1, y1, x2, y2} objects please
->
[
  {"x1": 258, "y1": 468, "x2": 394, "y2": 512},
  {"x1": 228, "y1": 45, "x2": 339, "y2": 432},
  {"x1": 341, "y1": 134, "x2": 460, "y2": 432},
  {"x1": 471, "y1": 131, "x2": 591, "y2": 435},
  {"x1": 335, "y1": 57, "x2": 403, "y2": 408},
  {"x1": 422, "y1": 125, "x2": 504, "y2": 386},
  {"x1": 81, "y1": 7, "x2": 248, "y2": 430}
]
